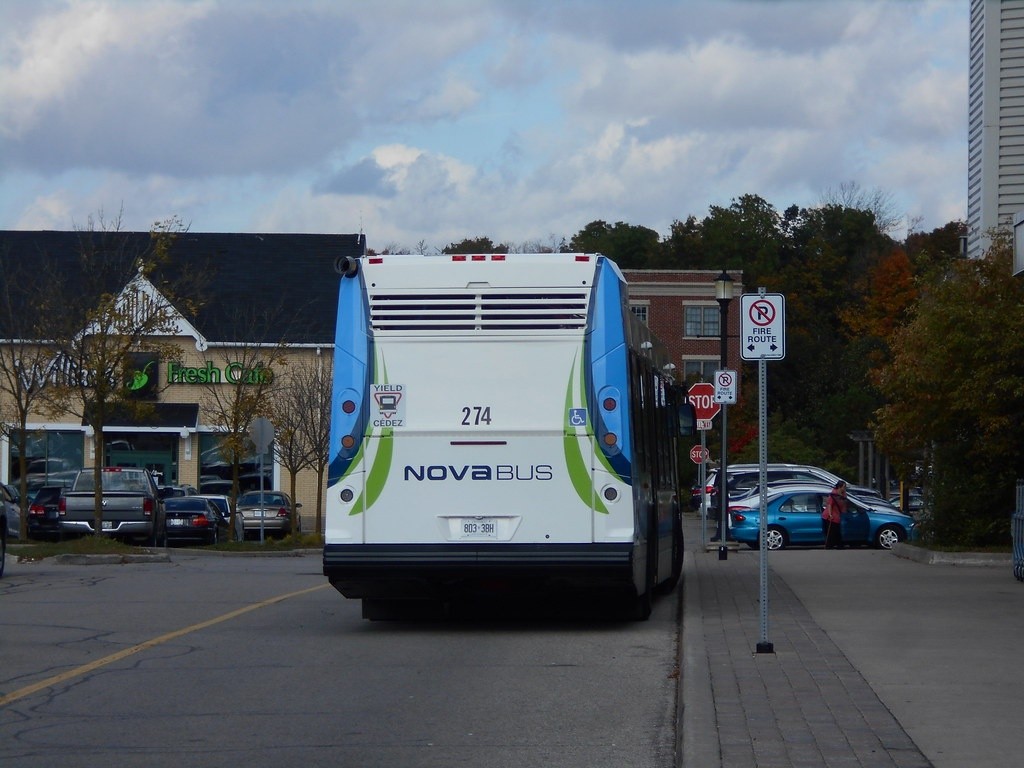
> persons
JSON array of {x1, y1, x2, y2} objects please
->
[
  {"x1": 710, "y1": 457, "x2": 731, "y2": 542},
  {"x1": 822, "y1": 480, "x2": 849, "y2": 549}
]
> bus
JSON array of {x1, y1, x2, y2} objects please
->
[{"x1": 322, "y1": 253, "x2": 698, "y2": 622}]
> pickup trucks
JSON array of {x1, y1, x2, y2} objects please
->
[{"x1": 57, "y1": 467, "x2": 174, "y2": 549}]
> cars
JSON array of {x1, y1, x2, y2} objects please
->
[
  {"x1": 163, "y1": 498, "x2": 231, "y2": 547},
  {"x1": 0, "y1": 483, "x2": 13, "y2": 577},
  {"x1": 727, "y1": 481, "x2": 913, "y2": 518},
  {"x1": 27, "y1": 457, "x2": 71, "y2": 477},
  {"x1": 106, "y1": 440, "x2": 135, "y2": 450},
  {"x1": 691, "y1": 469, "x2": 717, "y2": 508},
  {"x1": 227, "y1": 490, "x2": 302, "y2": 540},
  {"x1": 0, "y1": 482, "x2": 29, "y2": 537},
  {"x1": 184, "y1": 494, "x2": 232, "y2": 524},
  {"x1": 200, "y1": 473, "x2": 272, "y2": 494},
  {"x1": 729, "y1": 489, "x2": 920, "y2": 551},
  {"x1": 890, "y1": 495, "x2": 934, "y2": 511},
  {"x1": 26, "y1": 485, "x2": 72, "y2": 539}
]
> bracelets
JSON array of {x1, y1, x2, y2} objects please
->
[{"x1": 829, "y1": 514, "x2": 832, "y2": 517}]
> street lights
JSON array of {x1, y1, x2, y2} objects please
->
[{"x1": 712, "y1": 270, "x2": 736, "y2": 560}]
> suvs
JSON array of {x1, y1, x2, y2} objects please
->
[
  {"x1": 156, "y1": 484, "x2": 200, "y2": 498},
  {"x1": 707, "y1": 465, "x2": 882, "y2": 519},
  {"x1": 699, "y1": 466, "x2": 850, "y2": 517}
]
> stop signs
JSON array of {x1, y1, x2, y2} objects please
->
[
  {"x1": 685, "y1": 383, "x2": 721, "y2": 421},
  {"x1": 690, "y1": 445, "x2": 710, "y2": 464}
]
